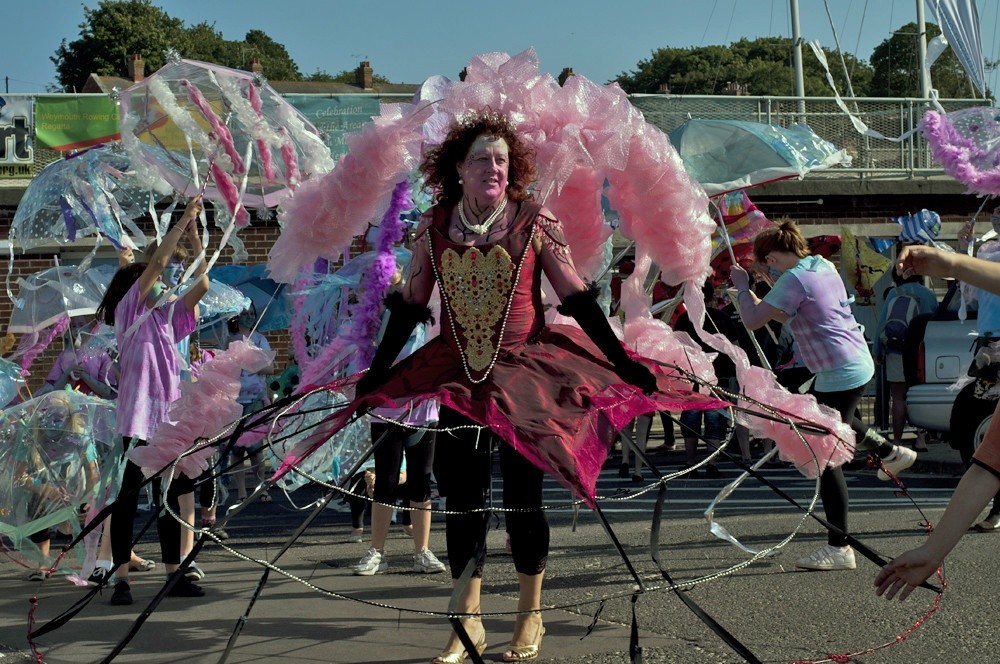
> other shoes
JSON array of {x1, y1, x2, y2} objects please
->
[
  {"x1": 632, "y1": 471, "x2": 645, "y2": 484},
  {"x1": 27, "y1": 571, "x2": 46, "y2": 581},
  {"x1": 911, "y1": 442, "x2": 928, "y2": 452},
  {"x1": 704, "y1": 465, "x2": 726, "y2": 479},
  {"x1": 130, "y1": 559, "x2": 156, "y2": 572},
  {"x1": 215, "y1": 529, "x2": 229, "y2": 540},
  {"x1": 677, "y1": 463, "x2": 699, "y2": 478},
  {"x1": 348, "y1": 532, "x2": 363, "y2": 543},
  {"x1": 619, "y1": 463, "x2": 629, "y2": 478}
]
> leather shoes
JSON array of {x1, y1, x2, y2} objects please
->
[{"x1": 975, "y1": 510, "x2": 1000, "y2": 533}]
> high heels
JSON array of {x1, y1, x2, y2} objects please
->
[
  {"x1": 430, "y1": 630, "x2": 487, "y2": 664},
  {"x1": 503, "y1": 620, "x2": 546, "y2": 661}
]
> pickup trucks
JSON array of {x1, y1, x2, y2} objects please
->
[{"x1": 905, "y1": 320, "x2": 1000, "y2": 469}]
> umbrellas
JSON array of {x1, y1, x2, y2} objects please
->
[
  {"x1": 0, "y1": 55, "x2": 417, "y2": 549},
  {"x1": 917, "y1": 105, "x2": 1000, "y2": 246},
  {"x1": 601, "y1": 112, "x2": 853, "y2": 265}
]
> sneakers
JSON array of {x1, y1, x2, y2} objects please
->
[
  {"x1": 110, "y1": 582, "x2": 132, "y2": 605},
  {"x1": 353, "y1": 547, "x2": 388, "y2": 575},
  {"x1": 412, "y1": 550, "x2": 446, "y2": 573},
  {"x1": 87, "y1": 568, "x2": 109, "y2": 588},
  {"x1": 165, "y1": 574, "x2": 206, "y2": 597},
  {"x1": 795, "y1": 545, "x2": 857, "y2": 570},
  {"x1": 877, "y1": 444, "x2": 918, "y2": 480}
]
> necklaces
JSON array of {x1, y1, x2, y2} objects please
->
[{"x1": 458, "y1": 193, "x2": 508, "y2": 235}]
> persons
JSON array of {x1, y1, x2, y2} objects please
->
[
  {"x1": 91, "y1": 194, "x2": 212, "y2": 606},
  {"x1": 957, "y1": 221, "x2": 1000, "y2": 355},
  {"x1": 871, "y1": 243, "x2": 999, "y2": 604},
  {"x1": 728, "y1": 217, "x2": 919, "y2": 572},
  {"x1": 615, "y1": 256, "x2": 864, "y2": 489},
  {"x1": 358, "y1": 104, "x2": 661, "y2": 664},
  {"x1": 16, "y1": 393, "x2": 101, "y2": 582},
  {"x1": 350, "y1": 267, "x2": 446, "y2": 577},
  {"x1": 871, "y1": 262, "x2": 939, "y2": 455}
]
